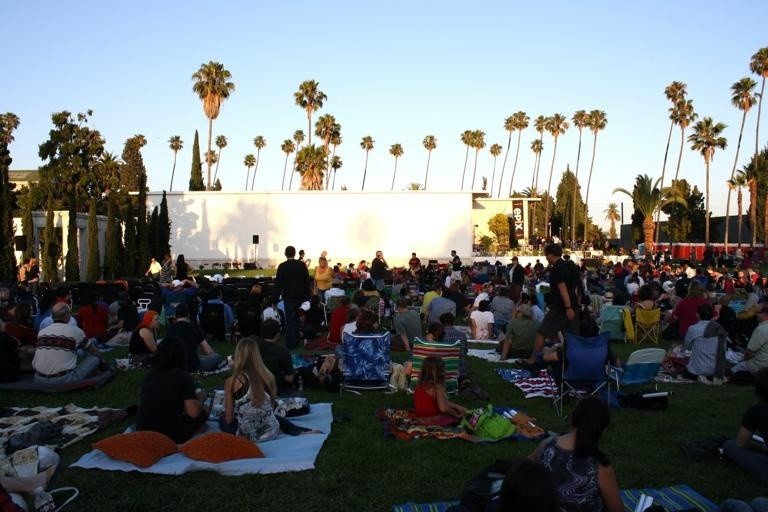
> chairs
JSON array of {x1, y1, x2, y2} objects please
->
[
  {"x1": 599, "y1": 303, "x2": 631, "y2": 344},
  {"x1": 198, "y1": 304, "x2": 226, "y2": 341},
  {"x1": 548, "y1": 345, "x2": 611, "y2": 420},
  {"x1": 633, "y1": 307, "x2": 661, "y2": 344},
  {"x1": 320, "y1": 296, "x2": 349, "y2": 325},
  {"x1": 229, "y1": 303, "x2": 262, "y2": 344},
  {"x1": 339, "y1": 330, "x2": 391, "y2": 391},
  {"x1": 359, "y1": 295, "x2": 380, "y2": 324},
  {"x1": 406, "y1": 335, "x2": 462, "y2": 395}
]
